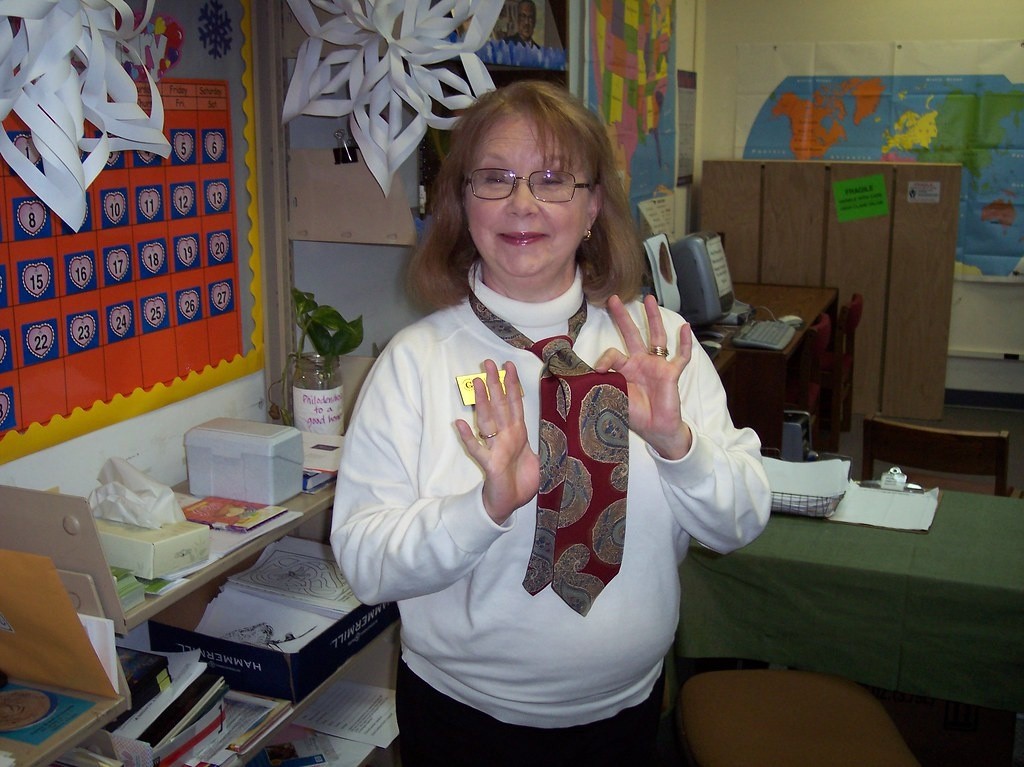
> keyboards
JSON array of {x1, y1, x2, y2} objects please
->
[{"x1": 732, "y1": 319, "x2": 796, "y2": 350}]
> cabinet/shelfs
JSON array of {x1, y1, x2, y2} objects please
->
[
  {"x1": 1, "y1": 477, "x2": 402, "y2": 767},
  {"x1": 702, "y1": 160, "x2": 961, "y2": 421}
]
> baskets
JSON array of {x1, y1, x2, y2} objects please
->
[{"x1": 769, "y1": 490, "x2": 846, "y2": 518}]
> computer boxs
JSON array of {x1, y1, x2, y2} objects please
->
[{"x1": 780, "y1": 410, "x2": 813, "y2": 462}]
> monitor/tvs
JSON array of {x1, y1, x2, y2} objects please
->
[{"x1": 668, "y1": 229, "x2": 735, "y2": 338}]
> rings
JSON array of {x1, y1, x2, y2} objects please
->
[
  {"x1": 648, "y1": 345, "x2": 669, "y2": 357},
  {"x1": 479, "y1": 431, "x2": 498, "y2": 439}
]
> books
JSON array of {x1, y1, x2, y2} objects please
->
[
  {"x1": 181, "y1": 496, "x2": 288, "y2": 533},
  {"x1": 52, "y1": 614, "x2": 295, "y2": 767},
  {"x1": 302, "y1": 472, "x2": 337, "y2": 495}
]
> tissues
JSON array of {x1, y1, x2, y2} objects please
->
[{"x1": 86, "y1": 455, "x2": 212, "y2": 582}]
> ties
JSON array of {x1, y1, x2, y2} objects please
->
[{"x1": 469, "y1": 292, "x2": 631, "y2": 617}]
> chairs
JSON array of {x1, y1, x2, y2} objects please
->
[
  {"x1": 785, "y1": 292, "x2": 863, "y2": 453},
  {"x1": 862, "y1": 412, "x2": 1024, "y2": 499},
  {"x1": 679, "y1": 667, "x2": 921, "y2": 767}
]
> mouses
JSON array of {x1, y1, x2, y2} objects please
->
[{"x1": 777, "y1": 316, "x2": 804, "y2": 327}]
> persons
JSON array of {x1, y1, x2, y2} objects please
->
[{"x1": 330, "y1": 80, "x2": 772, "y2": 767}]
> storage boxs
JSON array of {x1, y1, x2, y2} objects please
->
[{"x1": 184, "y1": 418, "x2": 303, "y2": 505}]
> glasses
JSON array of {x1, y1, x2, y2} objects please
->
[{"x1": 466, "y1": 168, "x2": 589, "y2": 203}]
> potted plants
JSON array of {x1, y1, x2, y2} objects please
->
[{"x1": 269, "y1": 287, "x2": 364, "y2": 436}]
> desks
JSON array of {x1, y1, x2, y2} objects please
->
[
  {"x1": 674, "y1": 481, "x2": 1024, "y2": 712},
  {"x1": 705, "y1": 282, "x2": 838, "y2": 408}
]
